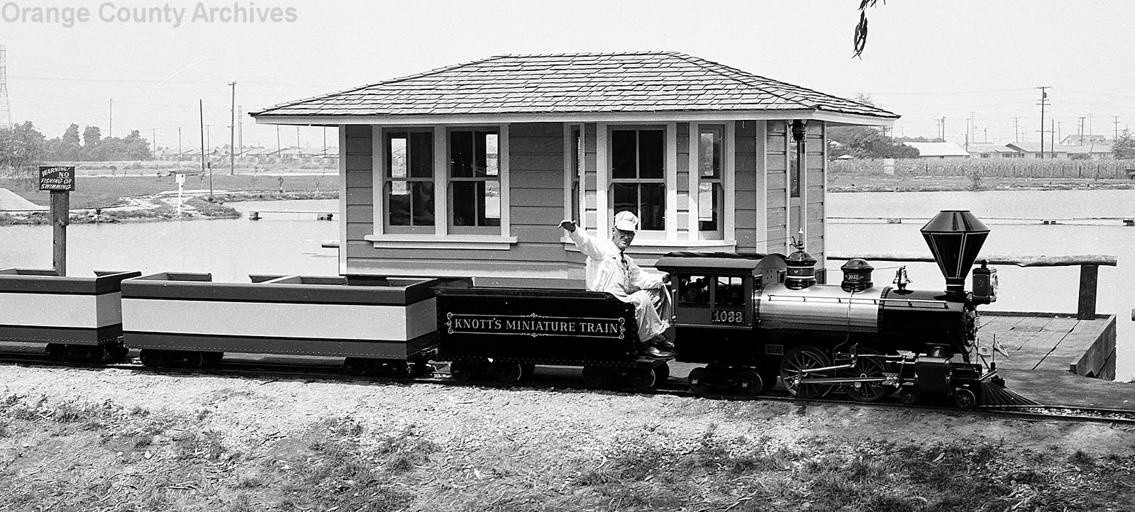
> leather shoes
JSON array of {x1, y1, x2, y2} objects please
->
[
  {"x1": 653, "y1": 339, "x2": 675, "y2": 350},
  {"x1": 643, "y1": 347, "x2": 674, "y2": 360}
]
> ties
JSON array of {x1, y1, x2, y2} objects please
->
[{"x1": 620, "y1": 251, "x2": 625, "y2": 263}]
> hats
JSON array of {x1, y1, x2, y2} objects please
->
[{"x1": 615, "y1": 210, "x2": 639, "y2": 234}]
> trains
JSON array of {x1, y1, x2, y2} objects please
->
[{"x1": 0, "y1": 208, "x2": 1045, "y2": 419}]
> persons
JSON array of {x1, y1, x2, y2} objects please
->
[{"x1": 557, "y1": 209, "x2": 675, "y2": 352}]
